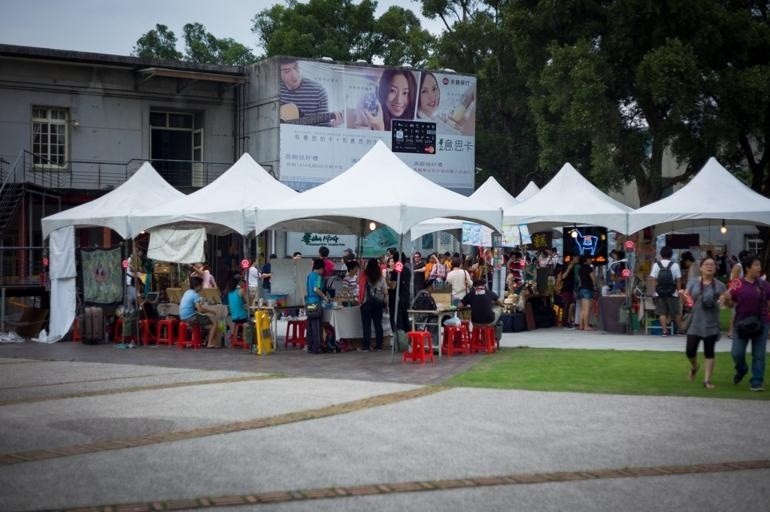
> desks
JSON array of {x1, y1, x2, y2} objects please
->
[
  {"x1": 154, "y1": 301, "x2": 229, "y2": 344},
  {"x1": 599, "y1": 292, "x2": 628, "y2": 334},
  {"x1": 321, "y1": 304, "x2": 392, "y2": 350},
  {"x1": 641, "y1": 294, "x2": 676, "y2": 335},
  {"x1": 247, "y1": 303, "x2": 304, "y2": 352},
  {"x1": 407, "y1": 306, "x2": 469, "y2": 357}
]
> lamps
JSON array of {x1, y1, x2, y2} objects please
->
[
  {"x1": 719, "y1": 219, "x2": 727, "y2": 233},
  {"x1": 570, "y1": 224, "x2": 582, "y2": 239},
  {"x1": 68, "y1": 120, "x2": 80, "y2": 129},
  {"x1": 368, "y1": 221, "x2": 377, "y2": 232}
]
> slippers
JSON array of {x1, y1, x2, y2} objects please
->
[
  {"x1": 702, "y1": 379, "x2": 715, "y2": 389},
  {"x1": 689, "y1": 363, "x2": 700, "y2": 381}
]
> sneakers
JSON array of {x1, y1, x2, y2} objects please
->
[
  {"x1": 662, "y1": 329, "x2": 669, "y2": 336},
  {"x1": 734, "y1": 368, "x2": 747, "y2": 384},
  {"x1": 748, "y1": 384, "x2": 766, "y2": 391}
]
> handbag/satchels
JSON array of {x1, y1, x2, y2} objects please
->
[
  {"x1": 733, "y1": 315, "x2": 763, "y2": 339},
  {"x1": 305, "y1": 302, "x2": 322, "y2": 319},
  {"x1": 373, "y1": 288, "x2": 386, "y2": 306},
  {"x1": 414, "y1": 292, "x2": 437, "y2": 310}
]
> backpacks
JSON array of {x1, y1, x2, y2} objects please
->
[{"x1": 655, "y1": 261, "x2": 677, "y2": 298}]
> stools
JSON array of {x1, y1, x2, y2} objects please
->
[
  {"x1": 158, "y1": 318, "x2": 179, "y2": 349},
  {"x1": 401, "y1": 330, "x2": 434, "y2": 365},
  {"x1": 134, "y1": 318, "x2": 154, "y2": 348},
  {"x1": 469, "y1": 324, "x2": 496, "y2": 355},
  {"x1": 111, "y1": 318, "x2": 133, "y2": 346},
  {"x1": 284, "y1": 317, "x2": 308, "y2": 348},
  {"x1": 232, "y1": 320, "x2": 256, "y2": 351}
]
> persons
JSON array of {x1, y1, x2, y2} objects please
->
[
  {"x1": 722, "y1": 255, "x2": 770, "y2": 392},
  {"x1": 651, "y1": 246, "x2": 751, "y2": 336},
  {"x1": 179, "y1": 277, "x2": 222, "y2": 348},
  {"x1": 679, "y1": 255, "x2": 727, "y2": 389},
  {"x1": 359, "y1": 69, "x2": 417, "y2": 131},
  {"x1": 279, "y1": 58, "x2": 345, "y2": 127},
  {"x1": 224, "y1": 276, "x2": 257, "y2": 348},
  {"x1": 416, "y1": 71, "x2": 441, "y2": 120},
  {"x1": 245, "y1": 246, "x2": 337, "y2": 354},
  {"x1": 341, "y1": 248, "x2": 506, "y2": 352},
  {"x1": 126, "y1": 245, "x2": 149, "y2": 313},
  {"x1": 183, "y1": 263, "x2": 216, "y2": 288},
  {"x1": 503, "y1": 249, "x2": 628, "y2": 330}
]
